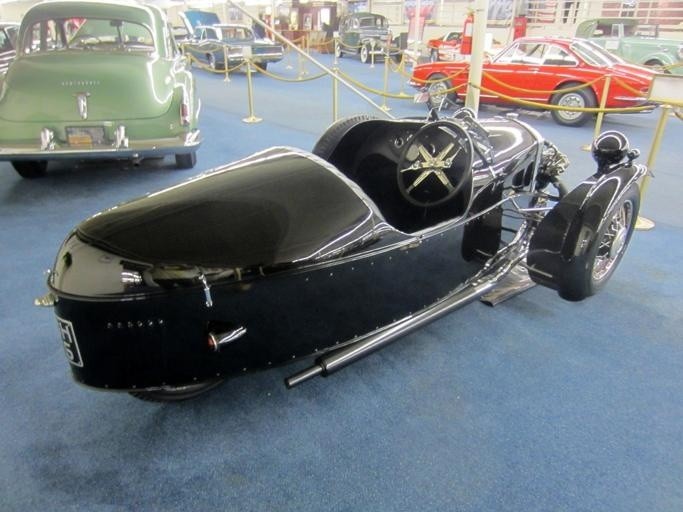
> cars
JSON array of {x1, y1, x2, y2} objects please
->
[
  {"x1": 412, "y1": 16, "x2": 683, "y2": 126},
  {"x1": 334, "y1": 12, "x2": 403, "y2": 65}
]
